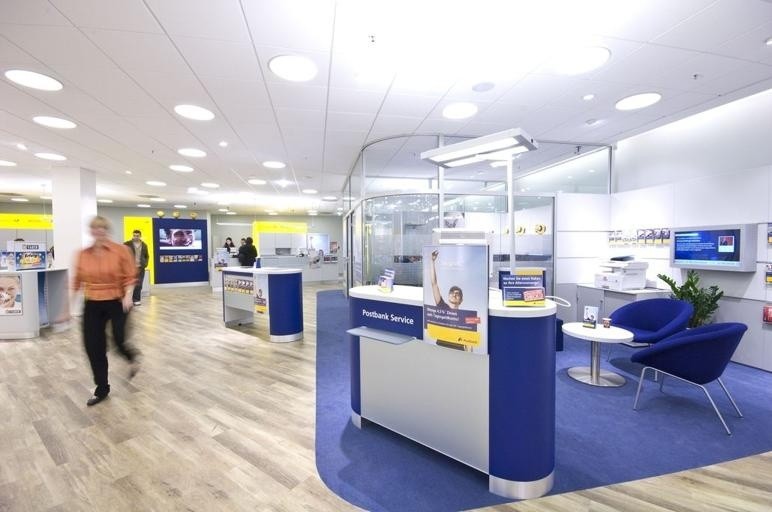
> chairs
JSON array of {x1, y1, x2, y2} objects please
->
[{"x1": 608, "y1": 299, "x2": 745, "y2": 438}]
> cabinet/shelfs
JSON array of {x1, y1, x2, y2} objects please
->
[
  {"x1": 347, "y1": 284, "x2": 557, "y2": 498},
  {"x1": 210, "y1": 253, "x2": 341, "y2": 295},
  {"x1": 218, "y1": 265, "x2": 303, "y2": 343}
]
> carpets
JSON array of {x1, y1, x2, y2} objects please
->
[{"x1": 316, "y1": 286, "x2": 772, "y2": 512}]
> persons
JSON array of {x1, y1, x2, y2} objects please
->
[
  {"x1": 239, "y1": 237, "x2": 257, "y2": 266},
  {"x1": 0, "y1": 276, "x2": 20, "y2": 314},
  {"x1": 56, "y1": 215, "x2": 143, "y2": 405},
  {"x1": 429, "y1": 250, "x2": 474, "y2": 352},
  {"x1": 223, "y1": 237, "x2": 234, "y2": 253},
  {"x1": 123, "y1": 229, "x2": 149, "y2": 306},
  {"x1": 171, "y1": 229, "x2": 193, "y2": 246},
  {"x1": 237, "y1": 238, "x2": 245, "y2": 252},
  {"x1": 585, "y1": 308, "x2": 595, "y2": 321}
]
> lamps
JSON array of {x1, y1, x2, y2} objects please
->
[{"x1": 419, "y1": 128, "x2": 543, "y2": 171}]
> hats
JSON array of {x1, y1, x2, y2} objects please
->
[{"x1": 247, "y1": 237, "x2": 252, "y2": 240}]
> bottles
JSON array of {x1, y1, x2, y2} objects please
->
[{"x1": 254, "y1": 256, "x2": 262, "y2": 269}]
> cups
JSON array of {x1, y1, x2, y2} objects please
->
[{"x1": 603, "y1": 317, "x2": 612, "y2": 328}]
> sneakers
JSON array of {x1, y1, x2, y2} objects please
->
[
  {"x1": 85, "y1": 395, "x2": 101, "y2": 405},
  {"x1": 133, "y1": 302, "x2": 140, "y2": 305}
]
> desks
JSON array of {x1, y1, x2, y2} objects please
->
[{"x1": 0, "y1": 266, "x2": 77, "y2": 340}]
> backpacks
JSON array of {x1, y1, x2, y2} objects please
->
[{"x1": 238, "y1": 245, "x2": 252, "y2": 262}]
